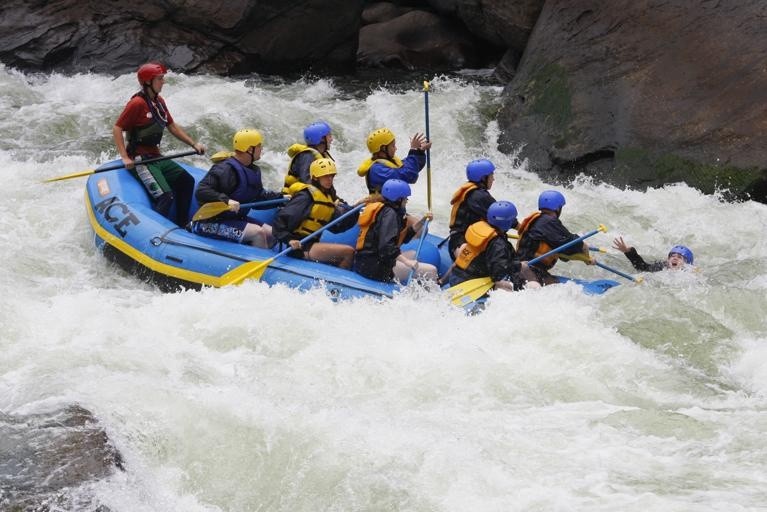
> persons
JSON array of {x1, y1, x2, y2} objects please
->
[
  {"x1": 353, "y1": 178, "x2": 437, "y2": 293},
  {"x1": 272, "y1": 158, "x2": 369, "y2": 273},
  {"x1": 449, "y1": 200, "x2": 530, "y2": 301},
  {"x1": 362, "y1": 127, "x2": 432, "y2": 195},
  {"x1": 516, "y1": 190, "x2": 598, "y2": 284},
  {"x1": 447, "y1": 159, "x2": 521, "y2": 260},
  {"x1": 611, "y1": 236, "x2": 693, "y2": 272},
  {"x1": 190, "y1": 128, "x2": 291, "y2": 251},
  {"x1": 112, "y1": 62, "x2": 205, "y2": 218},
  {"x1": 282, "y1": 121, "x2": 347, "y2": 205}
]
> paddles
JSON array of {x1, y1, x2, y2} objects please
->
[
  {"x1": 556, "y1": 250, "x2": 643, "y2": 284},
  {"x1": 214, "y1": 203, "x2": 367, "y2": 285},
  {"x1": 444, "y1": 223, "x2": 608, "y2": 307},
  {"x1": 192, "y1": 198, "x2": 289, "y2": 220}
]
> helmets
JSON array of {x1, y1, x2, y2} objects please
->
[
  {"x1": 486, "y1": 200, "x2": 518, "y2": 229},
  {"x1": 303, "y1": 120, "x2": 332, "y2": 146},
  {"x1": 668, "y1": 245, "x2": 694, "y2": 265},
  {"x1": 137, "y1": 64, "x2": 168, "y2": 87},
  {"x1": 465, "y1": 159, "x2": 496, "y2": 183},
  {"x1": 309, "y1": 158, "x2": 338, "y2": 181},
  {"x1": 232, "y1": 130, "x2": 265, "y2": 153},
  {"x1": 381, "y1": 178, "x2": 412, "y2": 202},
  {"x1": 366, "y1": 128, "x2": 396, "y2": 154},
  {"x1": 538, "y1": 190, "x2": 566, "y2": 211}
]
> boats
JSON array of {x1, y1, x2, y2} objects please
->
[
  {"x1": 41, "y1": 81, "x2": 646, "y2": 349},
  {"x1": 85, "y1": 161, "x2": 621, "y2": 317}
]
